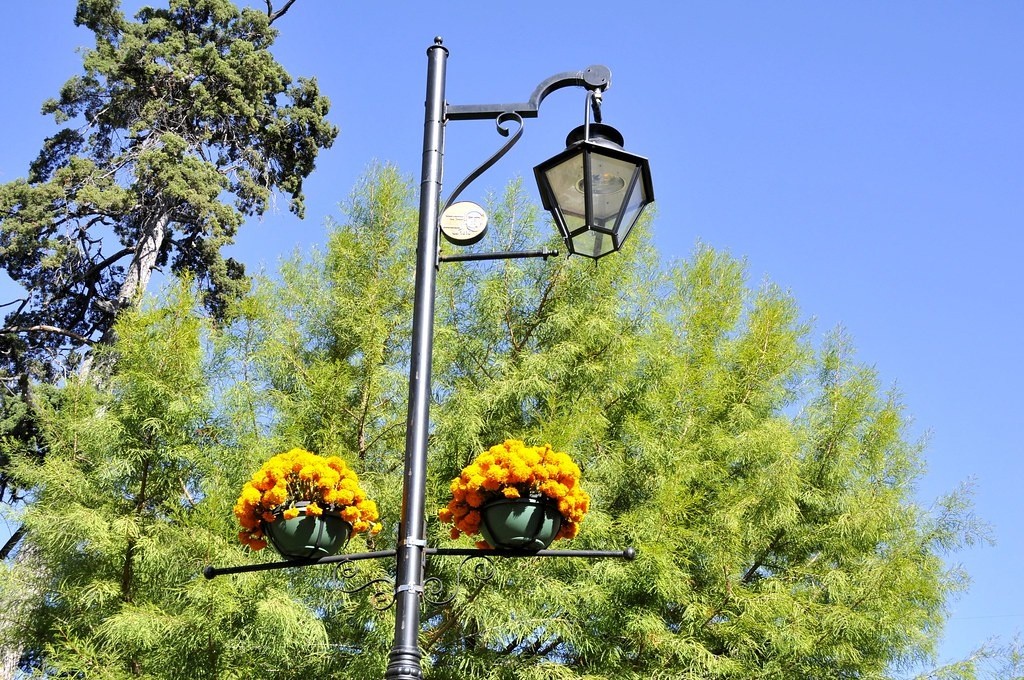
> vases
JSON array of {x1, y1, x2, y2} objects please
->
[
  {"x1": 477, "y1": 493, "x2": 562, "y2": 550},
  {"x1": 262, "y1": 501, "x2": 354, "y2": 561}
]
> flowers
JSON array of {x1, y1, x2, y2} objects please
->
[
  {"x1": 438, "y1": 439, "x2": 590, "y2": 549},
  {"x1": 232, "y1": 447, "x2": 382, "y2": 552}
]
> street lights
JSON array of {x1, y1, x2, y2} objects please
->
[{"x1": 384, "y1": 35, "x2": 658, "y2": 680}]
